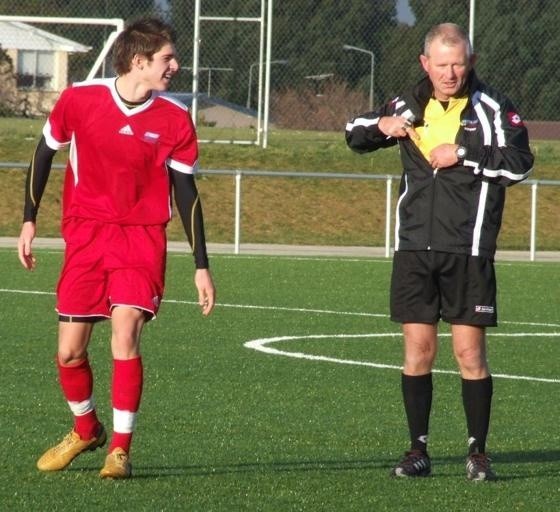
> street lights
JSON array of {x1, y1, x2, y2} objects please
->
[
  {"x1": 342, "y1": 43, "x2": 377, "y2": 112},
  {"x1": 246, "y1": 59, "x2": 288, "y2": 110}
]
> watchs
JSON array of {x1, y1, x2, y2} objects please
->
[{"x1": 455, "y1": 145, "x2": 467, "y2": 167}]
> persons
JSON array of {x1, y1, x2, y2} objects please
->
[
  {"x1": 18, "y1": 20, "x2": 216, "y2": 480},
  {"x1": 344, "y1": 22, "x2": 534, "y2": 483}
]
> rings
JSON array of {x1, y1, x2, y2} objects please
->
[{"x1": 402, "y1": 124, "x2": 409, "y2": 130}]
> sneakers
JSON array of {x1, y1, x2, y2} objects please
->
[
  {"x1": 465, "y1": 451, "x2": 495, "y2": 481},
  {"x1": 98, "y1": 447, "x2": 131, "y2": 479},
  {"x1": 390, "y1": 450, "x2": 430, "y2": 478},
  {"x1": 36, "y1": 422, "x2": 106, "y2": 471}
]
